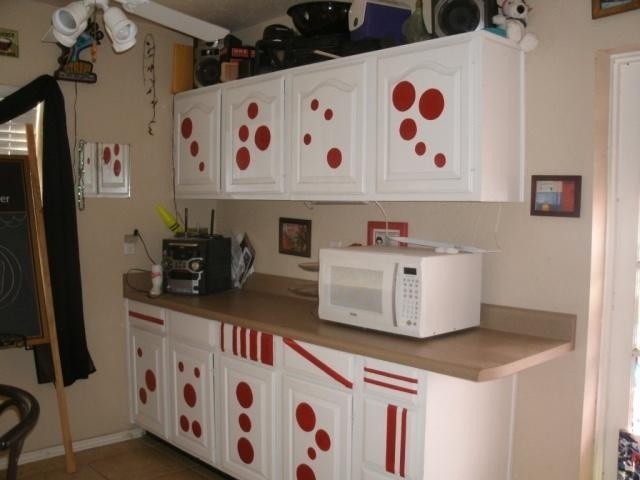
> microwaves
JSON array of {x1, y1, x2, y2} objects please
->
[{"x1": 318, "y1": 245, "x2": 482, "y2": 341}]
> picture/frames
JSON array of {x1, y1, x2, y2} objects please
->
[
  {"x1": 591, "y1": 0, "x2": 640, "y2": 20},
  {"x1": 278, "y1": 217, "x2": 312, "y2": 257},
  {"x1": 530, "y1": 174, "x2": 582, "y2": 217},
  {"x1": 367, "y1": 221, "x2": 409, "y2": 248}
]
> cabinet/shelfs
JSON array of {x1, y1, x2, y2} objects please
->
[
  {"x1": 83, "y1": 143, "x2": 129, "y2": 196},
  {"x1": 353, "y1": 354, "x2": 517, "y2": 480},
  {"x1": 174, "y1": 82, "x2": 222, "y2": 199},
  {"x1": 222, "y1": 69, "x2": 288, "y2": 199},
  {"x1": 288, "y1": 50, "x2": 372, "y2": 204},
  {"x1": 126, "y1": 297, "x2": 216, "y2": 468},
  {"x1": 215, "y1": 320, "x2": 353, "y2": 480},
  {"x1": 372, "y1": 28, "x2": 525, "y2": 201}
]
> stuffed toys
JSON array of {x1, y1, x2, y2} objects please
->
[{"x1": 493, "y1": 0, "x2": 540, "y2": 53}]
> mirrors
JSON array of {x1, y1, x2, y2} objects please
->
[{"x1": 77, "y1": 140, "x2": 131, "y2": 199}]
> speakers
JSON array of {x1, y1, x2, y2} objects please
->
[
  {"x1": 421, "y1": 0, "x2": 498, "y2": 38},
  {"x1": 193, "y1": 38, "x2": 225, "y2": 88}
]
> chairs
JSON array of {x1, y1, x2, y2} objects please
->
[{"x1": 0, "y1": 384, "x2": 40, "y2": 480}]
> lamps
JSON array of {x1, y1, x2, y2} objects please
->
[{"x1": 51, "y1": 2, "x2": 138, "y2": 54}]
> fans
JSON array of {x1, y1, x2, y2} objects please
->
[{"x1": 41, "y1": 0, "x2": 231, "y2": 43}]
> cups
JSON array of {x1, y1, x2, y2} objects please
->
[{"x1": 149, "y1": 264, "x2": 164, "y2": 295}]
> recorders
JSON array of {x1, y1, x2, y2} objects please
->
[{"x1": 160, "y1": 232, "x2": 231, "y2": 294}]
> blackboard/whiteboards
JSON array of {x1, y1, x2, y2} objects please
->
[{"x1": 0, "y1": 155, "x2": 48, "y2": 349}]
